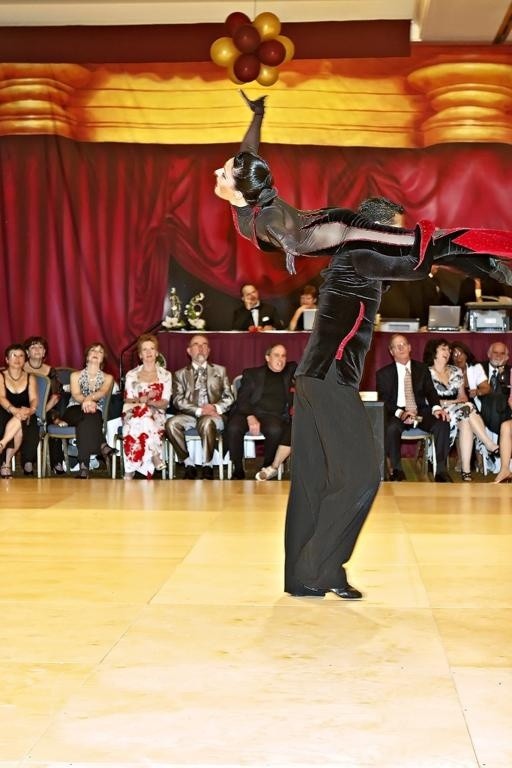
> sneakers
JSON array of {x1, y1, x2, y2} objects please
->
[{"x1": 255, "y1": 466, "x2": 278, "y2": 481}]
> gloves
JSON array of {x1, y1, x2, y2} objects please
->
[
  {"x1": 265, "y1": 224, "x2": 300, "y2": 276},
  {"x1": 239, "y1": 89, "x2": 268, "y2": 115}
]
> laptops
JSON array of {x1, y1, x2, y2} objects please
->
[
  {"x1": 427, "y1": 306, "x2": 461, "y2": 331},
  {"x1": 304, "y1": 308, "x2": 317, "y2": 331}
]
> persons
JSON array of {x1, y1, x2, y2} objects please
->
[
  {"x1": 22, "y1": 336, "x2": 67, "y2": 476},
  {"x1": 0, "y1": 344, "x2": 38, "y2": 479},
  {"x1": 63, "y1": 343, "x2": 120, "y2": 480},
  {"x1": 288, "y1": 284, "x2": 318, "y2": 331},
  {"x1": 283, "y1": 195, "x2": 440, "y2": 602},
  {"x1": 166, "y1": 335, "x2": 235, "y2": 480},
  {"x1": 374, "y1": 265, "x2": 512, "y2": 484},
  {"x1": 214, "y1": 90, "x2": 512, "y2": 298},
  {"x1": 226, "y1": 344, "x2": 301, "y2": 481},
  {"x1": 231, "y1": 284, "x2": 284, "y2": 332},
  {"x1": 122, "y1": 334, "x2": 172, "y2": 480}
]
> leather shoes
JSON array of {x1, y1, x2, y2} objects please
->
[
  {"x1": 230, "y1": 470, "x2": 245, "y2": 479},
  {"x1": 201, "y1": 466, "x2": 214, "y2": 480},
  {"x1": 156, "y1": 460, "x2": 167, "y2": 471},
  {"x1": 304, "y1": 580, "x2": 362, "y2": 598},
  {"x1": 124, "y1": 471, "x2": 135, "y2": 480},
  {"x1": 435, "y1": 471, "x2": 454, "y2": 483},
  {"x1": 393, "y1": 469, "x2": 405, "y2": 481},
  {"x1": 185, "y1": 465, "x2": 197, "y2": 479}
]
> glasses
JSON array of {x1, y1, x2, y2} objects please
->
[
  {"x1": 452, "y1": 351, "x2": 464, "y2": 357},
  {"x1": 391, "y1": 342, "x2": 408, "y2": 348},
  {"x1": 190, "y1": 344, "x2": 209, "y2": 350},
  {"x1": 8, "y1": 353, "x2": 25, "y2": 358}
]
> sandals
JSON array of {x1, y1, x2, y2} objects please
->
[{"x1": 1, "y1": 462, "x2": 13, "y2": 479}]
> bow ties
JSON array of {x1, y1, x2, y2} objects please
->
[{"x1": 251, "y1": 306, "x2": 261, "y2": 310}]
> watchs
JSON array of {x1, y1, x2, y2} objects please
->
[{"x1": 7, "y1": 404, "x2": 14, "y2": 414}]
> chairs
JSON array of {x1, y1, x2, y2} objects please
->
[
  {"x1": 112, "y1": 426, "x2": 167, "y2": 481},
  {"x1": 401, "y1": 429, "x2": 431, "y2": 476},
  {"x1": 168, "y1": 428, "x2": 226, "y2": 479},
  {"x1": 11, "y1": 374, "x2": 51, "y2": 478},
  {"x1": 227, "y1": 375, "x2": 284, "y2": 481},
  {"x1": 54, "y1": 367, "x2": 78, "y2": 385},
  {"x1": 42, "y1": 382, "x2": 114, "y2": 479},
  {"x1": 429, "y1": 433, "x2": 487, "y2": 478}
]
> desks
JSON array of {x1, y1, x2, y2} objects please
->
[{"x1": 158, "y1": 331, "x2": 512, "y2": 458}]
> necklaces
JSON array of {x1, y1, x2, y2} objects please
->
[
  {"x1": 28, "y1": 359, "x2": 42, "y2": 369},
  {"x1": 7, "y1": 368, "x2": 23, "y2": 382}
]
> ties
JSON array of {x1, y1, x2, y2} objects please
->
[
  {"x1": 195, "y1": 367, "x2": 208, "y2": 407},
  {"x1": 490, "y1": 370, "x2": 498, "y2": 392},
  {"x1": 404, "y1": 367, "x2": 418, "y2": 413}
]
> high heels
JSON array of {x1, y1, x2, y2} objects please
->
[
  {"x1": 80, "y1": 468, "x2": 88, "y2": 479},
  {"x1": 54, "y1": 466, "x2": 65, "y2": 475},
  {"x1": 461, "y1": 470, "x2": 472, "y2": 482},
  {"x1": 488, "y1": 472, "x2": 512, "y2": 484},
  {"x1": 487, "y1": 448, "x2": 501, "y2": 463},
  {"x1": 100, "y1": 444, "x2": 118, "y2": 460},
  {"x1": 23, "y1": 465, "x2": 33, "y2": 476}
]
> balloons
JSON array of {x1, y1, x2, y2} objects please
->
[{"x1": 210, "y1": 9, "x2": 295, "y2": 84}]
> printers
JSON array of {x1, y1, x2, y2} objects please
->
[{"x1": 465, "y1": 310, "x2": 508, "y2": 332}]
